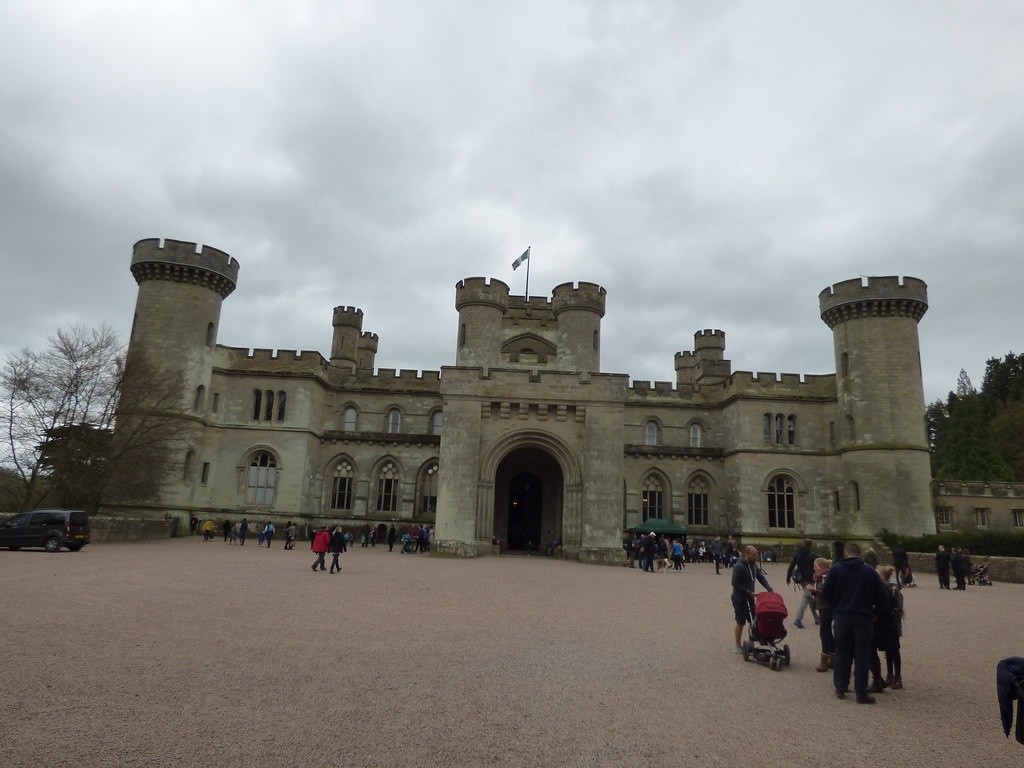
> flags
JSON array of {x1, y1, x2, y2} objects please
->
[{"x1": 512, "y1": 250, "x2": 528, "y2": 271}]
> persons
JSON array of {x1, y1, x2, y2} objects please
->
[
  {"x1": 892, "y1": 541, "x2": 911, "y2": 587},
  {"x1": 732, "y1": 545, "x2": 773, "y2": 654},
  {"x1": 545, "y1": 540, "x2": 558, "y2": 556},
  {"x1": 15, "y1": 515, "x2": 29, "y2": 523},
  {"x1": 935, "y1": 545, "x2": 990, "y2": 591},
  {"x1": 387, "y1": 524, "x2": 396, "y2": 552},
  {"x1": 628, "y1": 532, "x2": 742, "y2": 574},
  {"x1": 310, "y1": 525, "x2": 356, "y2": 574},
  {"x1": 221, "y1": 518, "x2": 248, "y2": 546},
  {"x1": 493, "y1": 536, "x2": 497, "y2": 545},
  {"x1": 409, "y1": 524, "x2": 434, "y2": 554},
  {"x1": 203, "y1": 519, "x2": 215, "y2": 542},
  {"x1": 786, "y1": 540, "x2": 902, "y2": 704},
  {"x1": 528, "y1": 541, "x2": 534, "y2": 556},
  {"x1": 256, "y1": 521, "x2": 275, "y2": 548},
  {"x1": 284, "y1": 521, "x2": 296, "y2": 550},
  {"x1": 191, "y1": 514, "x2": 198, "y2": 535},
  {"x1": 361, "y1": 522, "x2": 377, "y2": 548}
]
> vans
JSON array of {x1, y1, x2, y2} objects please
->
[{"x1": 0, "y1": 509, "x2": 91, "y2": 553}]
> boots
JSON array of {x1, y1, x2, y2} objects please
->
[
  {"x1": 816, "y1": 652, "x2": 830, "y2": 672},
  {"x1": 826, "y1": 653, "x2": 835, "y2": 669}
]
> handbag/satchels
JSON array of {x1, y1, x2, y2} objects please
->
[{"x1": 791, "y1": 569, "x2": 803, "y2": 584}]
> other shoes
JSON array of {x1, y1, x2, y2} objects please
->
[
  {"x1": 337, "y1": 567, "x2": 341, "y2": 572},
  {"x1": 883, "y1": 674, "x2": 895, "y2": 687},
  {"x1": 793, "y1": 619, "x2": 804, "y2": 628},
  {"x1": 892, "y1": 675, "x2": 903, "y2": 689},
  {"x1": 311, "y1": 565, "x2": 317, "y2": 571},
  {"x1": 815, "y1": 621, "x2": 820, "y2": 624},
  {"x1": 733, "y1": 647, "x2": 744, "y2": 654},
  {"x1": 321, "y1": 568, "x2": 326, "y2": 571},
  {"x1": 856, "y1": 695, "x2": 876, "y2": 704},
  {"x1": 329, "y1": 571, "x2": 335, "y2": 574},
  {"x1": 867, "y1": 677, "x2": 887, "y2": 693},
  {"x1": 835, "y1": 691, "x2": 845, "y2": 699}
]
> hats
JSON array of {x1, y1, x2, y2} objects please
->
[
  {"x1": 650, "y1": 531, "x2": 656, "y2": 538},
  {"x1": 815, "y1": 558, "x2": 833, "y2": 571}
]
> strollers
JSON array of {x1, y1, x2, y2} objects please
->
[
  {"x1": 900, "y1": 562, "x2": 918, "y2": 589},
  {"x1": 743, "y1": 592, "x2": 791, "y2": 671},
  {"x1": 401, "y1": 534, "x2": 416, "y2": 554},
  {"x1": 967, "y1": 561, "x2": 993, "y2": 587}
]
